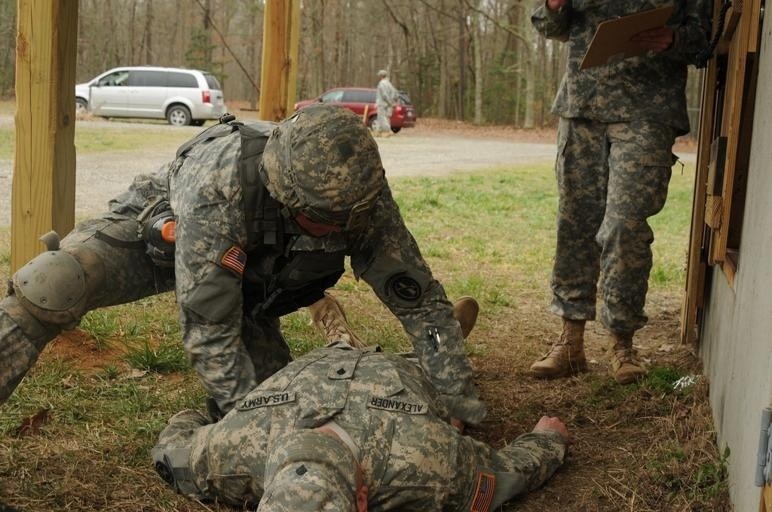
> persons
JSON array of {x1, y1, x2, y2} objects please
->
[
  {"x1": 529, "y1": 0, "x2": 714, "y2": 385},
  {"x1": 151, "y1": 292, "x2": 572, "y2": 512},
  {"x1": 372, "y1": 70, "x2": 398, "y2": 137},
  {"x1": 0, "y1": 99, "x2": 492, "y2": 436}
]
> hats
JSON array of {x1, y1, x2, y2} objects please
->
[
  {"x1": 258, "y1": 431, "x2": 361, "y2": 512},
  {"x1": 377, "y1": 70, "x2": 387, "y2": 77}
]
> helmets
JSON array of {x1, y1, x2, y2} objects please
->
[{"x1": 262, "y1": 104, "x2": 385, "y2": 228}]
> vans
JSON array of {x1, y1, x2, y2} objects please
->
[
  {"x1": 294, "y1": 87, "x2": 417, "y2": 132},
  {"x1": 75, "y1": 66, "x2": 224, "y2": 126}
]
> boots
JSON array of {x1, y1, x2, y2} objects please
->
[
  {"x1": 528, "y1": 321, "x2": 586, "y2": 379},
  {"x1": 451, "y1": 296, "x2": 478, "y2": 338},
  {"x1": 310, "y1": 292, "x2": 364, "y2": 349},
  {"x1": 605, "y1": 333, "x2": 648, "y2": 386}
]
